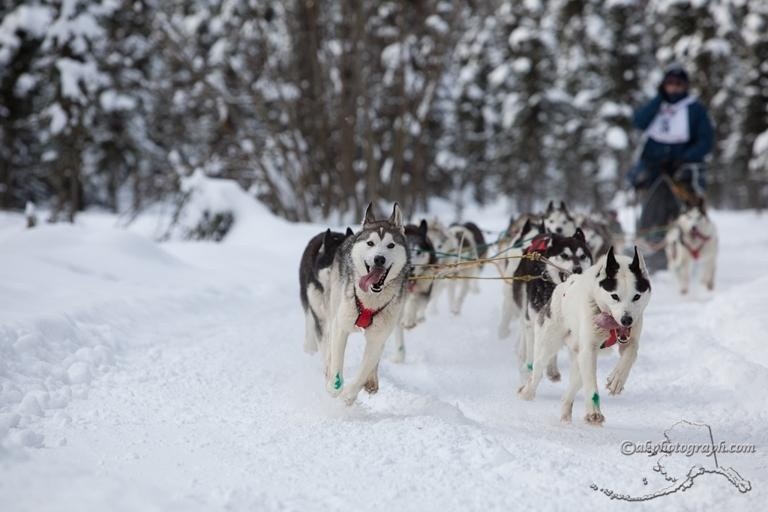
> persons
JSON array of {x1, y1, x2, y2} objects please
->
[{"x1": 626, "y1": 65, "x2": 717, "y2": 274}]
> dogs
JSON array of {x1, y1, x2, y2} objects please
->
[
  {"x1": 662, "y1": 197, "x2": 720, "y2": 295},
  {"x1": 510, "y1": 246, "x2": 653, "y2": 427},
  {"x1": 500, "y1": 200, "x2": 626, "y2": 382},
  {"x1": 323, "y1": 199, "x2": 411, "y2": 407},
  {"x1": 390, "y1": 216, "x2": 488, "y2": 364},
  {"x1": 297, "y1": 226, "x2": 354, "y2": 356}
]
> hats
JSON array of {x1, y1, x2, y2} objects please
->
[{"x1": 665, "y1": 69, "x2": 687, "y2": 85}]
